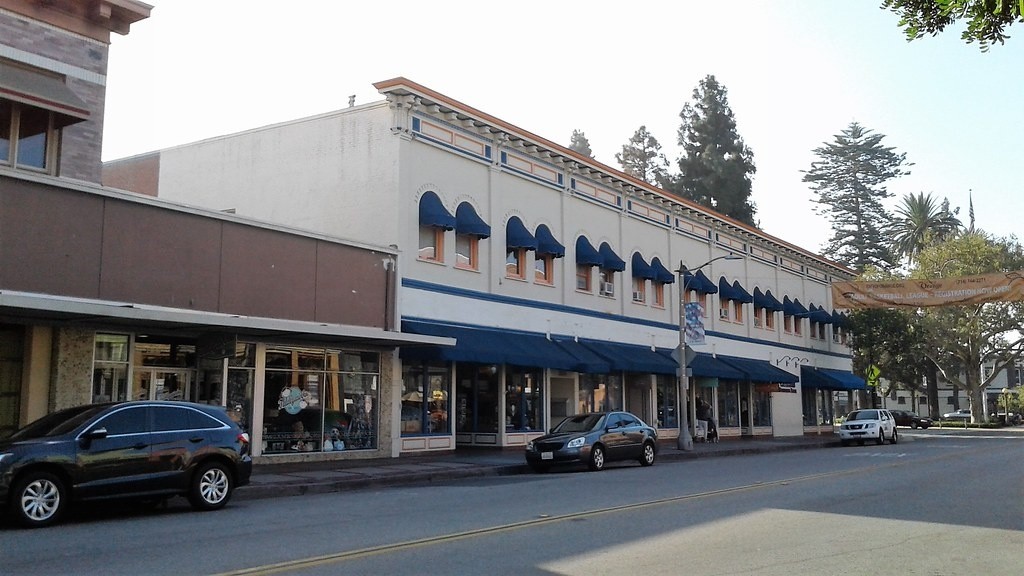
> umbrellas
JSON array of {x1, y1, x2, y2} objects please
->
[{"x1": 401, "y1": 391, "x2": 436, "y2": 402}]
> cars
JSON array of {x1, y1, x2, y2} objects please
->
[
  {"x1": 274, "y1": 408, "x2": 369, "y2": 448},
  {"x1": 525, "y1": 410, "x2": 660, "y2": 473},
  {"x1": 0, "y1": 401, "x2": 253, "y2": 528},
  {"x1": 943, "y1": 409, "x2": 973, "y2": 423}
]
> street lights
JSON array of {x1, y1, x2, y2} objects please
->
[
  {"x1": 674, "y1": 253, "x2": 744, "y2": 449},
  {"x1": 1001, "y1": 387, "x2": 1010, "y2": 424}
]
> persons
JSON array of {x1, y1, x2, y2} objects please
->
[
  {"x1": 343, "y1": 387, "x2": 371, "y2": 449},
  {"x1": 695, "y1": 392, "x2": 702, "y2": 409},
  {"x1": 741, "y1": 397, "x2": 748, "y2": 425},
  {"x1": 697, "y1": 399, "x2": 719, "y2": 442},
  {"x1": 507, "y1": 404, "x2": 520, "y2": 429}
]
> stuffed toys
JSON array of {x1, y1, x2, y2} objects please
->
[{"x1": 292, "y1": 421, "x2": 345, "y2": 451}]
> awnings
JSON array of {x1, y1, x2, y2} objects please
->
[
  {"x1": 397, "y1": 314, "x2": 799, "y2": 385},
  {"x1": 684, "y1": 269, "x2": 717, "y2": 295},
  {"x1": 632, "y1": 251, "x2": 674, "y2": 285},
  {"x1": 419, "y1": 191, "x2": 491, "y2": 241},
  {"x1": 506, "y1": 215, "x2": 565, "y2": 260},
  {"x1": 754, "y1": 287, "x2": 850, "y2": 331},
  {"x1": 576, "y1": 235, "x2": 626, "y2": 273},
  {"x1": 719, "y1": 276, "x2": 753, "y2": 304},
  {"x1": 801, "y1": 365, "x2": 874, "y2": 391}
]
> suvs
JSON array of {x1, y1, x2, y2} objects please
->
[
  {"x1": 889, "y1": 409, "x2": 933, "y2": 429},
  {"x1": 839, "y1": 408, "x2": 899, "y2": 445},
  {"x1": 657, "y1": 406, "x2": 674, "y2": 424}
]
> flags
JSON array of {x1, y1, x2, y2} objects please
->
[{"x1": 835, "y1": 391, "x2": 839, "y2": 396}]
